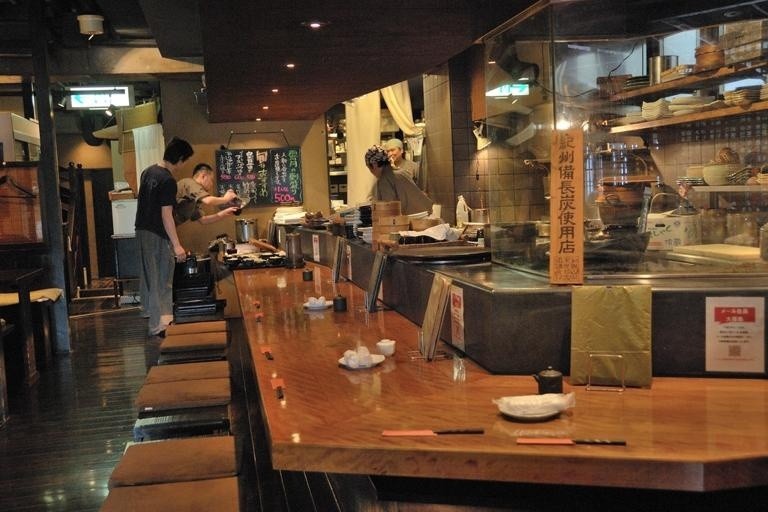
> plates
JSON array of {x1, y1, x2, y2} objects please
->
[
  {"x1": 676, "y1": 177, "x2": 705, "y2": 185},
  {"x1": 497, "y1": 395, "x2": 561, "y2": 423},
  {"x1": 623, "y1": 76, "x2": 649, "y2": 92},
  {"x1": 303, "y1": 300, "x2": 334, "y2": 310},
  {"x1": 640, "y1": 84, "x2": 768, "y2": 121},
  {"x1": 337, "y1": 353, "x2": 385, "y2": 369}
]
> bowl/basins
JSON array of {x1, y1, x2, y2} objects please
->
[{"x1": 702, "y1": 163, "x2": 743, "y2": 185}]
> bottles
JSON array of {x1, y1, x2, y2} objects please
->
[{"x1": 477, "y1": 227, "x2": 485, "y2": 249}]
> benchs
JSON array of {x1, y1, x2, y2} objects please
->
[{"x1": 0, "y1": 287, "x2": 63, "y2": 371}]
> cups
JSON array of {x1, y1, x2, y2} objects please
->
[
  {"x1": 333, "y1": 293, "x2": 347, "y2": 312},
  {"x1": 471, "y1": 208, "x2": 488, "y2": 224},
  {"x1": 687, "y1": 166, "x2": 704, "y2": 179},
  {"x1": 533, "y1": 365, "x2": 563, "y2": 396},
  {"x1": 303, "y1": 268, "x2": 313, "y2": 281},
  {"x1": 377, "y1": 338, "x2": 396, "y2": 357}
]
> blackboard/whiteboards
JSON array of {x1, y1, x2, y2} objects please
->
[{"x1": 214, "y1": 145, "x2": 304, "y2": 210}]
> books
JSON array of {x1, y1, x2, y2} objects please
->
[
  {"x1": 363, "y1": 249, "x2": 389, "y2": 313},
  {"x1": 264, "y1": 219, "x2": 277, "y2": 245},
  {"x1": 415, "y1": 271, "x2": 454, "y2": 365},
  {"x1": 330, "y1": 235, "x2": 345, "y2": 284}
]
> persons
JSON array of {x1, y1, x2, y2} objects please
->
[
  {"x1": 139, "y1": 162, "x2": 243, "y2": 320},
  {"x1": 134, "y1": 135, "x2": 195, "y2": 338},
  {"x1": 363, "y1": 143, "x2": 432, "y2": 216},
  {"x1": 364, "y1": 137, "x2": 422, "y2": 202}
]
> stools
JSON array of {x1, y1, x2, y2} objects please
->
[{"x1": 100, "y1": 315, "x2": 244, "y2": 512}]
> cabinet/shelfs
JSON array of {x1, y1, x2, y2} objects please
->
[{"x1": 605, "y1": 55, "x2": 768, "y2": 136}]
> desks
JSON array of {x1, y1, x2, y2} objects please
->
[{"x1": 1, "y1": 265, "x2": 47, "y2": 388}]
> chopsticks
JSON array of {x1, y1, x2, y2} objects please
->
[
  {"x1": 431, "y1": 428, "x2": 486, "y2": 436},
  {"x1": 571, "y1": 438, "x2": 626, "y2": 447},
  {"x1": 277, "y1": 385, "x2": 284, "y2": 399},
  {"x1": 255, "y1": 304, "x2": 273, "y2": 361}
]
